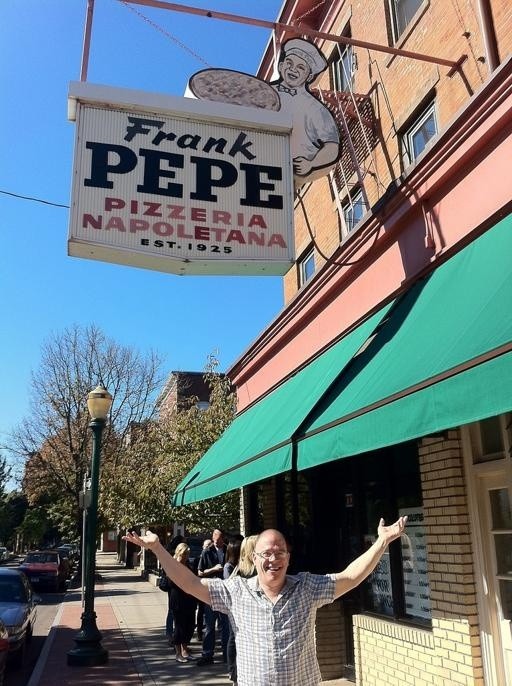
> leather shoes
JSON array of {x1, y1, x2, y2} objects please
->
[{"x1": 197, "y1": 624, "x2": 206, "y2": 640}]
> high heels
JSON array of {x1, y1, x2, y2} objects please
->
[{"x1": 165, "y1": 630, "x2": 174, "y2": 647}]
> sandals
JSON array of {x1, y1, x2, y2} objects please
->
[{"x1": 176, "y1": 654, "x2": 197, "y2": 663}]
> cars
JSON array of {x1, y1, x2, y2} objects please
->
[{"x1": 0, "y1": 543, "x2": 78, "y2": 685}]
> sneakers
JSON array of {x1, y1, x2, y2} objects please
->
[{"x1": 197, "y1": 656, "x2": 212, "y2": 666}]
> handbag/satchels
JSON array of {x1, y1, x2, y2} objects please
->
[{"x1": 159, "y1": 575, "x2": 170, "y2": 592}]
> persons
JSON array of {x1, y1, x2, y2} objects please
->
[
  {"x1": 270, "y1": 36, "x2": 341, "y2": 176},
  {"x1": 122, "y1": 515, "x2": 408, "y2": 686},
  {"x1": 159, "y1": 529, "x2": 259, "y2": 685}
]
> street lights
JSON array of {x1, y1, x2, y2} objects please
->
[{"x1": 64, "y1": 379, "x2": 111, "y2": 667}]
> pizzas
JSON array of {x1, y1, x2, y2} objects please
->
[{"x1": 191, "y1": 70, "x2": 280, "y2": 112}]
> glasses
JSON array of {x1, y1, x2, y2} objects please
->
[{"x1": 253, "y1": 550, "x2": 288, "y2": 560}]
